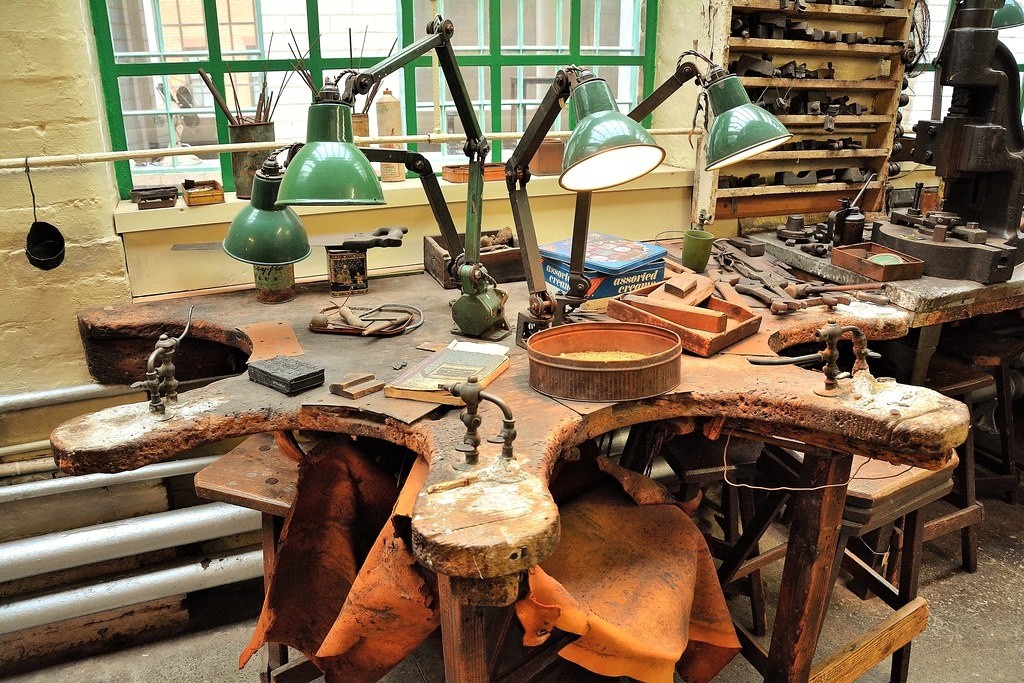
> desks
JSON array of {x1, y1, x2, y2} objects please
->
[
  {"x1": 748, "y1": 216, "x2": 1024, "y2": 600},
  {"x1": 49, "y1": 237, "x2": 971, "y2": 682}
]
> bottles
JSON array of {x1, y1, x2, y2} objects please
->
[{"x1": 375, "y1": 87, "x2": 406, "y2": 182}]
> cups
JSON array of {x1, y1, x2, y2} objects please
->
[{"x1": 681, "y1": 230, "x2": 714, "y2": 273}]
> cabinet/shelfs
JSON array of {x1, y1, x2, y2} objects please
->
[{"x1": 692, "y1": 0, "x2": 918, "y2": 224}]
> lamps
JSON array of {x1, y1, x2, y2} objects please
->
[
  {"x1": 553, "y1": 52, "x2": 795, "y2": 327},
  {"x1": 274, "y1": 14, "x2": 512, "y2": 341},
  {"x1": 504, "y1": 64, "x2": 668, "y2": 349},
  {"x1": 221, "y1": 139, "x2": 496, "y2": 309}
]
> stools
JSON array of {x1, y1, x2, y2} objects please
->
[
  {"x1": 865, "y1": 332, "x2": 984, "y2": 586},
  {"x1": 194, "y1": 432, "x2": 326, "y2": 682},
  {"x1": 716, "y1": 438, "x2": 959, "y2": 682},
  {"x1": 944, "y1": 309, "x2": 1024, "y2": 503},
  {"x1": 631, "y1": 423, "x2": 767, "y2": 636}
]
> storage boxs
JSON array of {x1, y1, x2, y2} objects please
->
[
  {"x1": 536, "y1": 230, "x2": 668, "y2": 298},
  {"x1": 830, "y1": 241, "x2": 924, "y2": 281},
  {"x1": 423, "y1": 229, "x2": 527, "y2": 289},
  {"x1": 529, "y1": 138, "x2": 564, "y2": 175},
  {"x1": 527, "y1": 321, "x2": 682, "y2": 402},
  {"x1": 130, "y1": 179, "x2": 225, "y2": 209},
  {"x1": 443, "y1": 162, "x2": 508, "y2": 183}
]
